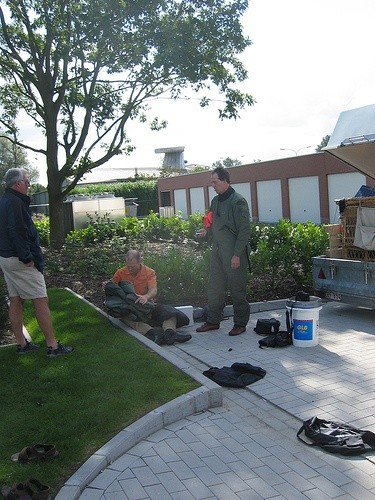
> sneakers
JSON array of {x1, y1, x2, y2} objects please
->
[
  {"x1": 15, "y1": 339, "x2": 41, "y2": 355},
  {"x1": 46, "y1": 340, "x2": 74, "y2": 357}
]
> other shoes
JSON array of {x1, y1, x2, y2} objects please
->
[
  {"x1": 196, "y1": 322, "x2": 220, "y2": 332},
  {"x1": 144, "y1": 328, "x2": 192, "y2": 346},
  {"x1": 228, "y1": 324, "x2": 246, "y2": 336}
]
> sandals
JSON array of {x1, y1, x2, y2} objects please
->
[
  {"x1": 1, "y1": 478, "x2": 51, "y2": 500},
  {"x1": 11, "y1": 442, "x2": 61, "y2": 464}
]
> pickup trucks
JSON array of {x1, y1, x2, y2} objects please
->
[{"x1": 311, "y1": 255, "x2": 375, "y2": 308}]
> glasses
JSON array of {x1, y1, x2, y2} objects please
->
[{"x1": 22, "y1": 179, "x2": 28, "y2": 185}]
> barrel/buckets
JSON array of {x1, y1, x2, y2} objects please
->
[{"x1": 285, "y1": 306, "x2": 323, "y2": 348}]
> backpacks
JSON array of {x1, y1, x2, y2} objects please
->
[
  {"x1": 297, "y1": 416, "x2": 375, "y2": 456},
  {"x1": 253, "y1": 318, "x2": 294, "y2": 350}
]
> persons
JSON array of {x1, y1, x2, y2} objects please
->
[
  {"x1": 0, "y1": 167, "x2": 73, "y2": 357},
  {"x1": 196, "y1": 167, "x2": 251, "y2": 336},
  {"x1": 102, "y1": 250, "x2": 192, "y2": 346}
]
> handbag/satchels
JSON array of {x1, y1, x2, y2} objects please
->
[
  {"x1": 295, "y1": 291, "x2": 309, "y2": 302},
  {"x1": 202, "y1": 302, "x2": 229, "y2": 322}
]
